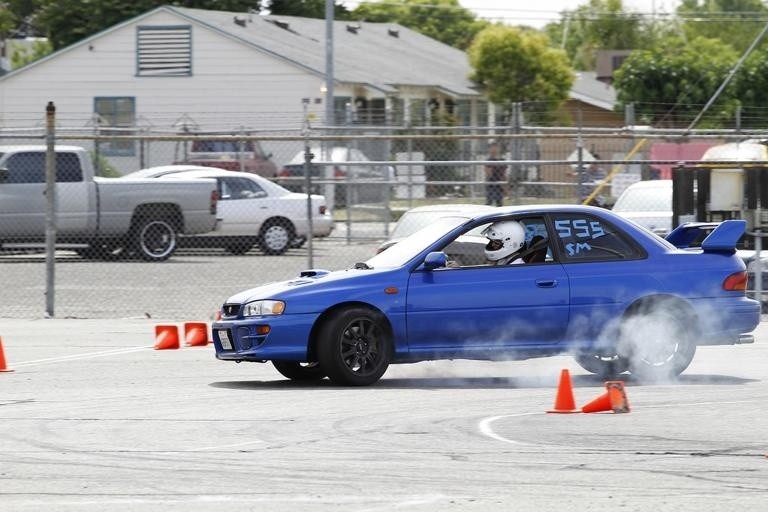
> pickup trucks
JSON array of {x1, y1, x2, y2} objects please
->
[
  {"x1": 176, "y1": 132, "x2": 276, "y2": 187},
  {"x1": 0, "y1": 136, "x2": 218, "y2": 263}
]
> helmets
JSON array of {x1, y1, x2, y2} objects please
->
[{"x1": 480, "y1": 220, "x2": 525, "y2": 261}]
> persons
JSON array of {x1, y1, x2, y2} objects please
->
[
  {"x1": 444, "y1": 220, "x2": 525, "y2": 269},
  {"x1": 567, "y1": 152, "x2": 607, "y2": 205},
  {"x1": 484, "y1": 140, "x2": 507, "y2": 208}
]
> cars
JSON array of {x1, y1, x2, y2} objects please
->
[
  {"x1": 281, "y1": 147, "x2": 394, "y2": 208},
  {"x1": 612, "y1": 180, "x2": 673, "y2": 239},
  {"x1": 79, "y1": 164, "x2": 332, "y2": 258}
]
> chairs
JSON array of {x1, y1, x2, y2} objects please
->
[{"x1": 524, "y1": 234, "x2": 549, "y2": 264}]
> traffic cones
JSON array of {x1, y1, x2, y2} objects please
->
[
  {"x1": 0, "y1": 337, "x2": 14, "y2": 373},
  {"x1": 582, "y1": 380, "x2": 631, "y2": 413},
  {"x1": 545, "y1": 369, "x2": 582, "y2": 414},
  {"x1": 184, "y1": 322, "x2": 208, "y2": 347},
  {"x1": 154, "y1": 326, "x2": 180, "y2": 349}
]
